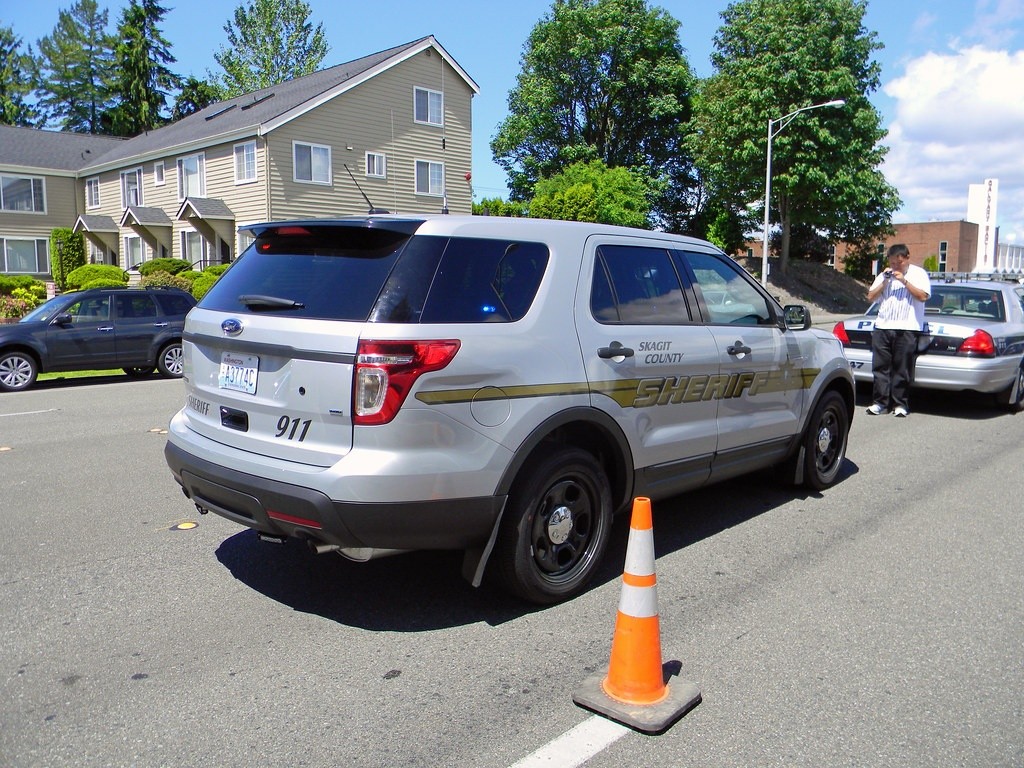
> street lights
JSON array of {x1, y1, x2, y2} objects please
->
[{"x1": 758, "y1": 98, "x2": 848, "y2": 296}]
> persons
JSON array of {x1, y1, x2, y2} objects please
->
[
  {"x1": 865, "y1": 244, "x2": 931, "y2": 416},
  {"x1": 988, "y1": 291, "x2": 998, "y2": 315}
]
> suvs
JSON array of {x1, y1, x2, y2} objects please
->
[
  {"x1": 0, "y1": 285, "x2": 199, "y2": 392},
  {"x1": 162, "y1": 209, "x2": 856, "y2": 610}
]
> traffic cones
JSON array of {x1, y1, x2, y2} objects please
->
[{"x1": 570, "y1": 495, "x2": 703, "y2": 735}]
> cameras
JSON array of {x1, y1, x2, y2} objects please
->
[{"x1": 883, "y1": 267, "x2": 894, "y2": 279}]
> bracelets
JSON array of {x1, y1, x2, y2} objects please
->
[{"x1": 903, "y1": 280, "x2": 908, "y2": 285}]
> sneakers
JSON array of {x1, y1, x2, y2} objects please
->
[
  {"x1": 895, "y1": 407, "x2": 907, "y2": 416},
  {"x1": 866, "y1": 403, "x2": 887, "y2": 414}
]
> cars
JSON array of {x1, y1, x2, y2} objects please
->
[{"x1": 831, "y1": 269, "x2": 1024, "y2": 414}]
